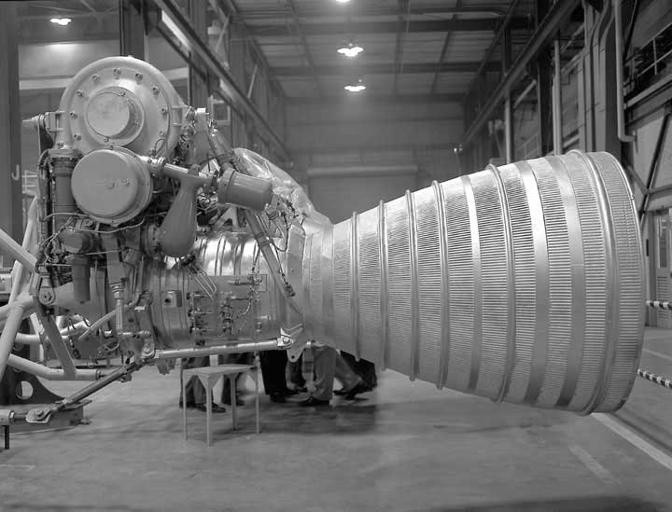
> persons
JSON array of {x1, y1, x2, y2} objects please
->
[{"x1": 179, "y1": 346, "x2": 377, "y2": 413}]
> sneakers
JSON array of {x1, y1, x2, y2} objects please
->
[
  {"x1": 221, "y1": 390, "x2": 246, "y2": 407},
  {"x1": 196, "y1": 402, "x2": 226, "y2": 414},
  {"x1": 296, "y1": 396, "x2": 331, "y2": 409},
  {"x1": 333, "y1": 381, "x2": 370, "y2": 400},
  {"x1": 178, "y1": 401, "x2": 196, "y2": 409},
  {"x1": 265, "y1": 384, "x2": 309, "y2": 406}
]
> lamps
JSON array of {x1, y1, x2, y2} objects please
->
[
  {"x1": 342, "y1": 73, "x2": 368, "y2": 95},
  {"x1": 334, "y1": 33, "x2": 368, "y2": 60}
]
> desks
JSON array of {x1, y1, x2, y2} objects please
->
[{"x1": 180, "y1": 363, "x2": 261, "y2": 449}]
